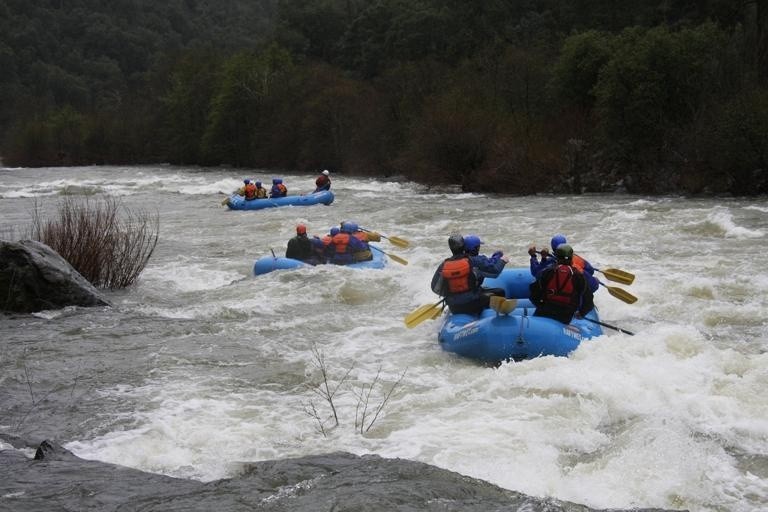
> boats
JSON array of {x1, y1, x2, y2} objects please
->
[
  {"x1": 224, "y1": 191, "x2": 335, "y2": 210},
  {"x1": 254, "y1": 246, "x2": 389, "y2": 274},
  {"x1": 437, "y1": 269, "x2": 601, "y2": 363}
]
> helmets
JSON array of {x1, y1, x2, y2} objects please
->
[
  {"x1": 296, "y1": 221, "x2": 358, "y2": 236},
  {"x1": 322, "y1": 170, "x2": 329, "y2": 175},
  {"x1": 244, "y1": 178, "x2": 282, "y2": 188},
  {"x1": 449, "y1": 235, "x2": 481, "y2": 251},
  {"x1": 551, "y1": 236, "x2": 573, "y2": 259}
]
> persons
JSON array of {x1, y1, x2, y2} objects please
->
[
  {"x1": 527, "y1": 243, "x2": 594, "y2": 325},
  {"x1": 527, "y1": 244, "x2": 599, "y2": 295},
  {"x1": 430, "y1": 232, "x2": 506, "y2": 313},
  {"x1": 461, "y1": 234, "x2": 504, "y2": 288},
  {"x1": 286, "y1": 221, "x2": 367, "y2": 267},
  {"x1": 238, "y1": 178, "x2": 287, "y2": 201},
  {"x1": 539, "y1": 234, "x2": 594, "y2": 277},
  {"x1": 313, "y1": 170, "x2": 330, "y2": 194}
]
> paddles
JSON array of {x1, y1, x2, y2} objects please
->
[
  {"x1": 595, "y1": 275, "x2": 638, "y2": 305},
  {"x1": 502, "y1": 300, "x2": 518, "y2": 316},
  {"x1": 364, "y1": 228, "x2": 409, "y2": 248},
  {"x1": 221, "y1": 193, "x2": 238, "y2": 207},
  {"x1": 596, "y1": 267, "x2": 635, "y2": 285},
  {"x1": 488, "y1": 295, "x2": 504, "y2": 316},
  {"x1": 371, "y1": 244, "x2": 409, "y2": 267},
  {"x1": 405, "y1": 297, "x2": 446, "y2": 328}
]
